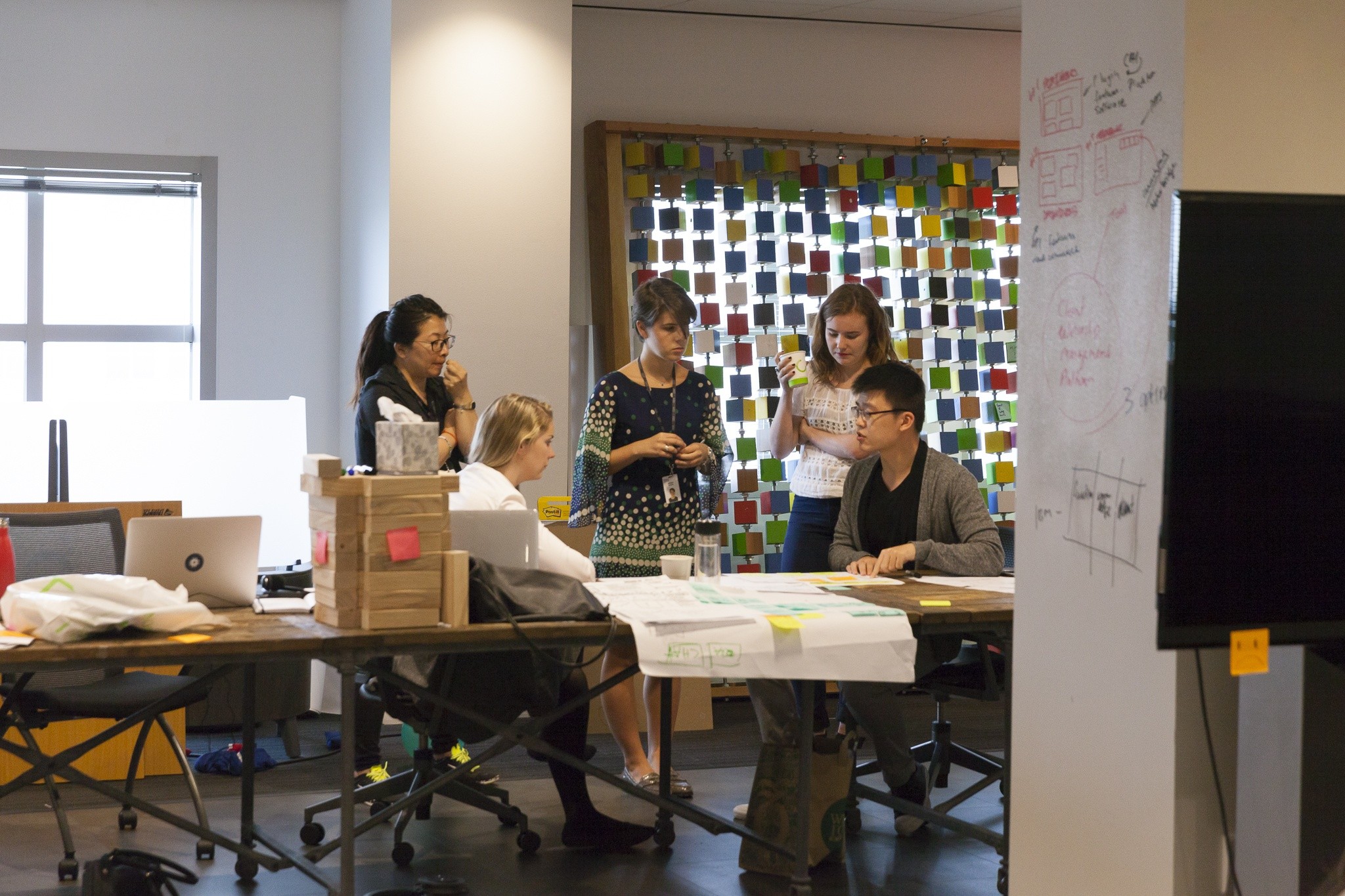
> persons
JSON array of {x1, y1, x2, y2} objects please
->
[
  {"x1": 668, "y1": 488, "x2": 678, "y2": 503},
  {"x1": 349, "y1": 295, "x2": 500, "y2": 807},
  {"x1": 568, "y1": 278, "x2": 732, "y2": 798},
  {"x1": 449, "y1": 394, "x2": 656, "y2": 849},
  {"x1": 733, "y1": 361, "x2": 1006, "y2": 834},
  {"x1": 767, "y1": 283, "x2": 902, "y2": 749}
]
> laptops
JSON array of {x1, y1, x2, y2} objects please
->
[{"x1": 121, "y1": 514, "x2": 262, "y2": 608}]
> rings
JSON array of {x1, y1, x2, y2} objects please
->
[
  {"x1": 775, "y1": 368, "x2": 780, "y2": 372},
  {"x1": 665, "y1": 446, "x2": 669, "y2": 452}
]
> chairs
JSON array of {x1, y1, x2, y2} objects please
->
[
  {"x1": 854, "y1": 528, "x2": 1015, "y2": 826},
  {"x1": 0, "y1": 507, "x2": 217, "y2": 882},
  {"x1": 299, "y1": 661, "x2": 543, "y2": 869}
]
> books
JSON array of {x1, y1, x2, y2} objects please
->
[{"x1": 253, "y1": 593, "x2": 316, "y2": 613}]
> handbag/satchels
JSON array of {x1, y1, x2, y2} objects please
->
[
  {"x1": 740, "y1": 730, "x2": 860, "y2": 876},
  {"x1": 0, "y1": 573, "x2": 234, "y2": 644}
]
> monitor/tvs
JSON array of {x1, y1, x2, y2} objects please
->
[{"x1": 1155, "y1": 192, "x2": 1345, "y2": 652}]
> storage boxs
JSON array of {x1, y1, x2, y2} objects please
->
[{"x1": 302, "y1": 419, "x2": 470, "y2": 631}]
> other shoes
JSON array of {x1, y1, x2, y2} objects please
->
[
  {"x1": 733, "y1": 803, "x2": 750, "y2": 819},
  {"x1": 620, "y1": 766, "x2": 659, "y2": 794},
  {"x1": 670, "y1": 771, "x2": 693, "y2": 799},
  {"x1": 895, "y1": 764, "x2": 929, "y2": 834}
]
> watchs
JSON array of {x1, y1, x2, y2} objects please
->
[{"x1": 454, "y1": 401, "x2": 476, "y2": 410}]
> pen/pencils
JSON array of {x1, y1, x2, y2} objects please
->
[{"x1": 348, "y1": 465, "x2": 376, "y2": 475}]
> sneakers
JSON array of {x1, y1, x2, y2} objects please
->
[
  {"x1": 353, "y1": 761, "x2": 393, "y2": 806},
  {"x1": 432, "y1": 743, "x2": 490, "y2": 781}
]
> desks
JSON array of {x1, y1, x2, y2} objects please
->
[{"x1": 2, "y1": 569, "x2": 1013, "y2": 888}]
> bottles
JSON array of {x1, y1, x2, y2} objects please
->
[
  {"x1": 0, "y1": 516, "x2": 15, "y2": 598},
  {"x1": 693, "y1": 519, "x2": 722, "y2": 595}
]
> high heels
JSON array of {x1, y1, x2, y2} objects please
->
[
  {"x1": 562, "y1": 808, "x2": 657, "y2": 850},
  {"x1": 528, "y1": 735, "x2": 597, "y2": 762}
]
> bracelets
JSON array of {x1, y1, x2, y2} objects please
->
[
  {"x1": 700, "y1": 439, "x2": 713, "y2": 466},
  {"x1": 438, "y1": 436, "x2": 452, "y2": 472},
  {"x1": 441, "y1": 430, "x2": 458, "y2": 446}
]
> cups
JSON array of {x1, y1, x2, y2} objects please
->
[
  {"x1": 659, "y1": 554, "x2": 693, "y2": 580},
  {"x1": 779, "y1": 351, "x2": 809, "y2": 389}
]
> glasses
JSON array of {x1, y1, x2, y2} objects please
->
[
  {"x1": 850, "y1": 406, "x2": 915, "y2": 421},
  {"x1": 410, "y1": 334, "x2": 456, "y2": 353}
]
